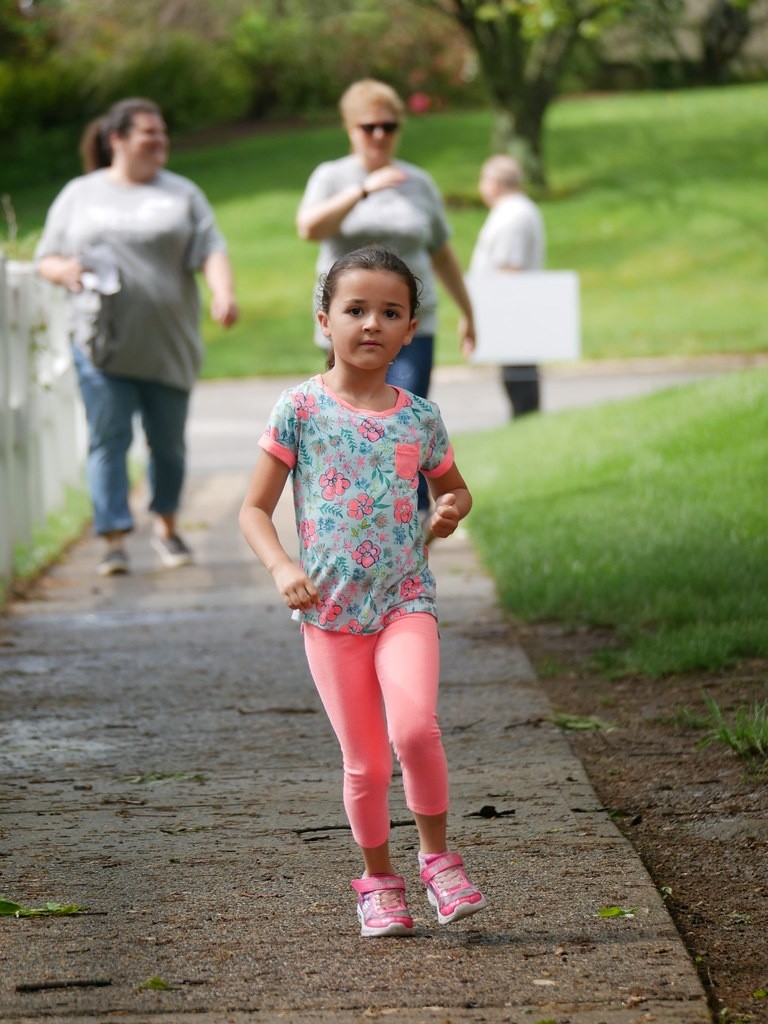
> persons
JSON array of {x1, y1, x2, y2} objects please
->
[
  {"x1": 295, "y1": 80, "x2": 477, "y2": 528},
  {"x1": 238, "y1": 247, "x2": 485, "y2": 939},
  {"x1": 460, "y1": 153, "x2": 545, "y2": 420},
  {"x1": 32, "y1": 100, "x2": 239, "y2": 581}
]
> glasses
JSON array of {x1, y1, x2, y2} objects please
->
[{"x1": 358, "y1": 121, "x2": 399, "y2": 136}]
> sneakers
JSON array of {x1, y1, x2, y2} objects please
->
[
  {"x1": 418, "y1": 851, "x2": 489, "y2": 927},
  {"x1": 95, "y1": 548, "x2": 132, "y2": 578},
  {"x1": 148, "y1": 529, "x2": 194, "y2": 572},
  {"x1": 350, "y1": 871, "x2": 415, "y2": 939}
]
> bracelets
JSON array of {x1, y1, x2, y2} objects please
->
[{"x1": 357, "y1": 180, "x2": 371, "y2": 201}]
